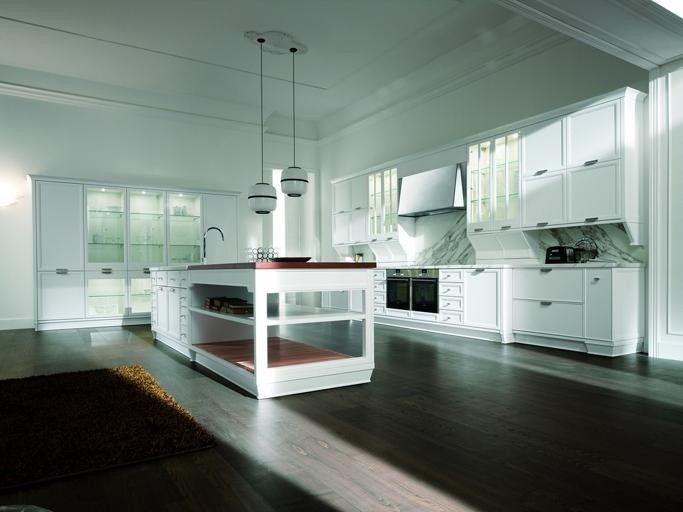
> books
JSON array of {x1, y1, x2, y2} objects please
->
[{"x1": 204, "y1": 297, "x2": 253, "y2": 314}]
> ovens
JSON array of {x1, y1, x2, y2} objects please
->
[{"x1": 385, "y1": 269, "x2": 438, "y2": 313}]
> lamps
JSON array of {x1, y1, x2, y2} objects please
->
[{"x1": 245, "y1": 31, "x2": 309, "y2": 214}]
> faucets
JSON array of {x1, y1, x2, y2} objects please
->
[{"x1": 202, "y1": 225, "x2": 225, "y2": 264}]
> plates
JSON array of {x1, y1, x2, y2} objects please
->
[{"x1": 267, "y1": 256, "x2": 310, "y2": 262}]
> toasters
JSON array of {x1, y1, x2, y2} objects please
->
[{"x1": 544, "y1": 245, "x2": 576, "y2": 263}]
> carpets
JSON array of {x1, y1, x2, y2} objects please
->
[{"x1": 1, "y1": 364, "x2": 218, "y2": 489}]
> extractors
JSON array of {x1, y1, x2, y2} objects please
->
[{"x1": 396, "y1": 164, "x2": 466, "y2": 217}]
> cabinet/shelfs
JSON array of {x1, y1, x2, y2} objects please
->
[
  {"x1": 519, "y1": 98, "x2": 621, "y2": 232},
  {"x1": 331, "y1": 174, "x2": 373, "y2": 245},
  {"x1": 512, "y1": 268, "x2": 646, "y2": 357},
  {"x1": 191, "y1": 268, "x2": 375, "y2": 400},
  {"x1": 374, "y1": 269, "x2": 386, "y2": 318},
  {"x1": 150, "y1": 271, "x2": 190, "y2": 359},
  {"x1": 35, "y1": 180, "x2": 239, "y2": 330},
  {"x1": 437, "y1": 269, "x2": 511, "y2": 344}
]
